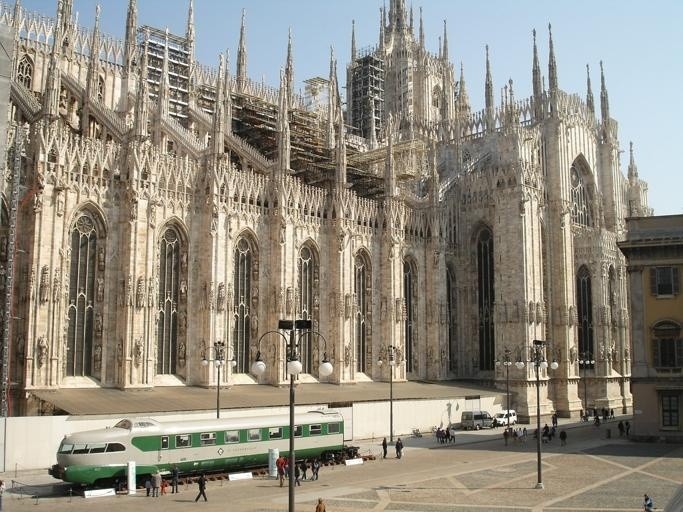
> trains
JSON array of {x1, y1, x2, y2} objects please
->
[{"x1": 49, "y1": 405, "x2": 345, "y2": 488}]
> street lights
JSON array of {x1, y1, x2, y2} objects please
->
[
  {"x1": 516, "y1": 340, "x2": 559, "y2": 490},
  {"x1": 374, "y1": 343, "x2": 407, "y2": 442},
  {"x1": 575, "y1": 351, "x2": 596, "y2": 419},
  {"x1": 493, "y1": 347, "x2": 518, "y2": 434},
  {"x1": 250, "y1": 316, "x2": 335, "y2": 512},
  {"x1": 202, "y1": 338, "x2": 237, "y2": 419}
]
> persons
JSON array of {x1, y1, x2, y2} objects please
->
[
  {"x1": 504, "y1": 413, "x2": 567, "y2": 447},
  {"x1": 436, "y1": 427, "x2": 456, "y2": 445},
  {"x1": 382, "y1": 437, "x2": 387, "y2": 458},
  {"x1": 316, "y1": 498, "x2": 326, "y2": 512},
  {"x1": 395, "y1": 438, "x2": 403, "y2": 459},
  {"x1": 643, "y1": 494, "x2": 654, "y2": 512},
  {"x1": 580, "y1": 407, "x2": 631, "y2": 436},
  {"x1": 276, "y1": 456, "x2": 320, "y2": 487},
  {"x1": 195, "y1": 473, "x2": 208, "y2": 502},
  {"x1": 145, "y1": 464, "x2": 179, "y2": 497}
]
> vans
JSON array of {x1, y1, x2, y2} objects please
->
[
  {"x1": 461, "y1": 410, "x2": 495, "y2": 431},
  {"x1": 493, "y1": 409, "x2": 518, "y2": 427}
]
